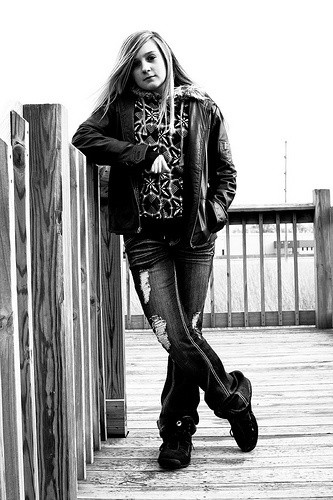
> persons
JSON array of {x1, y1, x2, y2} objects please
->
[{"x1": 70, "y1": 30, "x2": 259, "y2": 473}]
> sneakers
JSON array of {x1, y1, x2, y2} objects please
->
[
  {"x1": 214, "y1": 377, "x2": 258, "y2": 453},
  {"x1": 157, "y1": 434, "x2": 192, "y2": 469}
]
